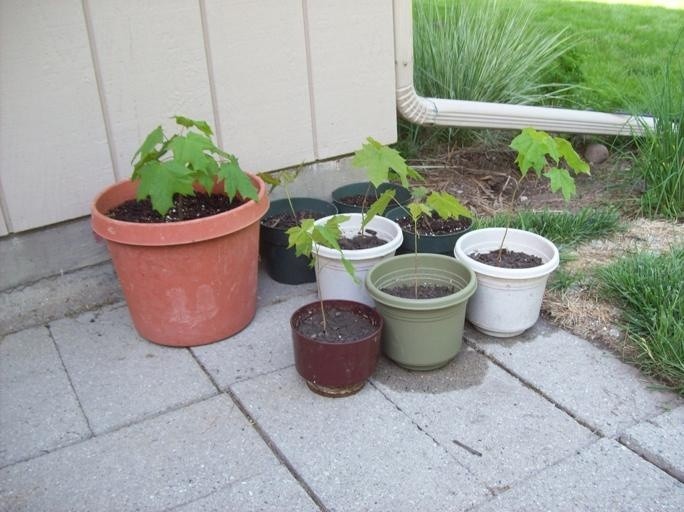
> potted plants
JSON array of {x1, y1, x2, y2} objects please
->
[
  {"x1": 262, "y1": 162, "x2": 339, "y2": 285},
  {"x1": 286, "y1": 214, "x2": 383, "y2": 398},
  {"x1": 386, "y1": 200, "x2": 473, "y2": 255},
  {"x1": 454, "y1": 129, "x2": 593, "y2": 337},
  {"x1": 331, "y1": 136, "x2": 425, "y2": 213},
  {"x1": 88, "y1": 111, "x2": 269, "y2": 349},
  {"x1": 308, "y1": 214, "x2": 403, "y2": 310},
  {"x1": 361, "y1": 187, "x2": 478, "y2": 372}
]
